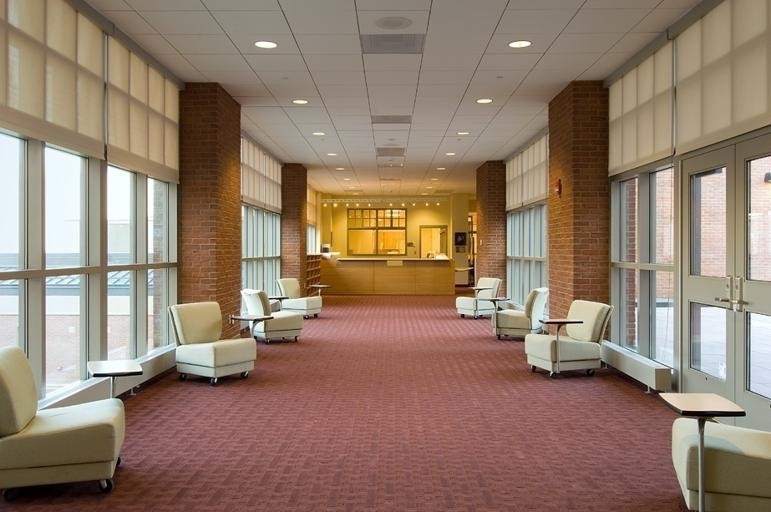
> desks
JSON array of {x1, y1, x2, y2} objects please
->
[{"x1": 660, "y1": 392, "x2": 745, "y2": 512}]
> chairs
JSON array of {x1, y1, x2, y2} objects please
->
[
  {"x1": 525, "y1": 301, "x2": 614, "y2": 377},
  {"x1": 455, "y1": 277, "x2": 502, "y2": 319},
  {"x1": 0, "y1": 346, "x2": 126, "y2": 494},
  {"x1": 168, "y1": 301, "x2": 256, "y2": 385},
  {"x1": 276, "y1": 278, "x2": 322, "y2": 318},
  {"x1": 495, "y1": 287, "x2": 549, "y2": 339},
  {"x1": 241, "y1": 289, "x2": 303, "y2": 343},
  {"x1": 672, "y1": 418, "x2": 771, "y2": 511}
]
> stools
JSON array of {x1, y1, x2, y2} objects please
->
[
  {"x1": 540, "y1": 320, "x2": 583, "y2": 374},
  {"x1": 478, "y1": 298, "x2": 509, "y2": 339},
  {"x1": 88, "y1": 359, "x2": 143, "y2": 398},
  {"x1": 269, "y1": 297, "x2": 289, "y2": 311},
  {"x1": 310, "y1": 285, "x2": 331, "y2": 296},
  {"x1": 232, "y1": 315, "x2": 274, "y2": 337},
  {"x1": 468, "y1": 287, "x2": 492, "y2": 318}
]
